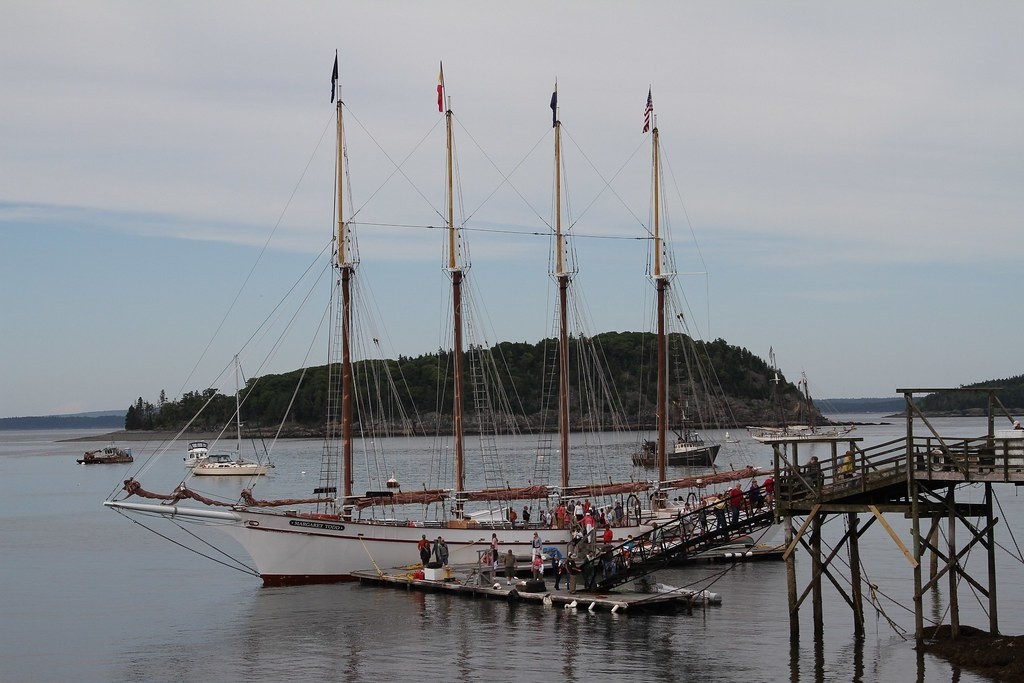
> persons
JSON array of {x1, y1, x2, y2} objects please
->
[
  {"x1": 647, "y1": 450, "x2": 855, "y2": 560},
  {"x1": 529, "y1": 532, "x2": 543, "y2": 564},
  {"x1": 510, "y1": 507, "x2": 517, "y2": 529},
  {"x1": 531, "y1": 553, "x2": 544, "y2": 582},
  {"x1": 540, "y1": 498, "x2": 634, "y2": 595},
  {"x1": 523, "y1": 506, "x2": 530, "y2": 530},
  {"x1": 503, "y1": 549, "x2": 518, "y2": 586},
  {"x1": 631, "y1": 495, "x2": 642, "y2": 526},
  {"x1": 492, "y1": 533, "x2": 499, "y2": 562},
  {"x1": 1012, "y1": 420, "x2": 1024, "y2": 430},
  {"x1": 417, "y1": 534, "x2": 449, "y2": 567}
]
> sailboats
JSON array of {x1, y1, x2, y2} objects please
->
[{"x1": 103, "y1": 48, "x2": 799, "y2": 588}]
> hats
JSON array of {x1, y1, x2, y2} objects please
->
[{"x1": 571, "y1": 553, "x2": 578, "y2": 559}]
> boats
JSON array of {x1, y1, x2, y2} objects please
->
[
  {"x1": 190, "y1": 354, "x2": 276, "y2": 475},
  {"x1": 75, "y1": 445, "x2": 134, "y2": 466},
  {"x1": 631, "y1": 392, "x2": 722, "y2": 468},
  {"x1": 182, "y1": 439, "x2": 209, "y2": 472},
  {"x1": 744, "y1": 345, "x2": 858, "y2": 438}
]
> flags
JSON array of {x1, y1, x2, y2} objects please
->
[
  {"x1": 437, "y1": 69, "x2": 444, "y2": 113},
  {"x1": 331, "y1": 55, "x2": 338, "y2": 103},
  {"x1": 549, "y1": 82, "x2": 557, "y2": 128},
  {"x1": 642, "y1": 87, "x2": 654, "y2": 133}
]
